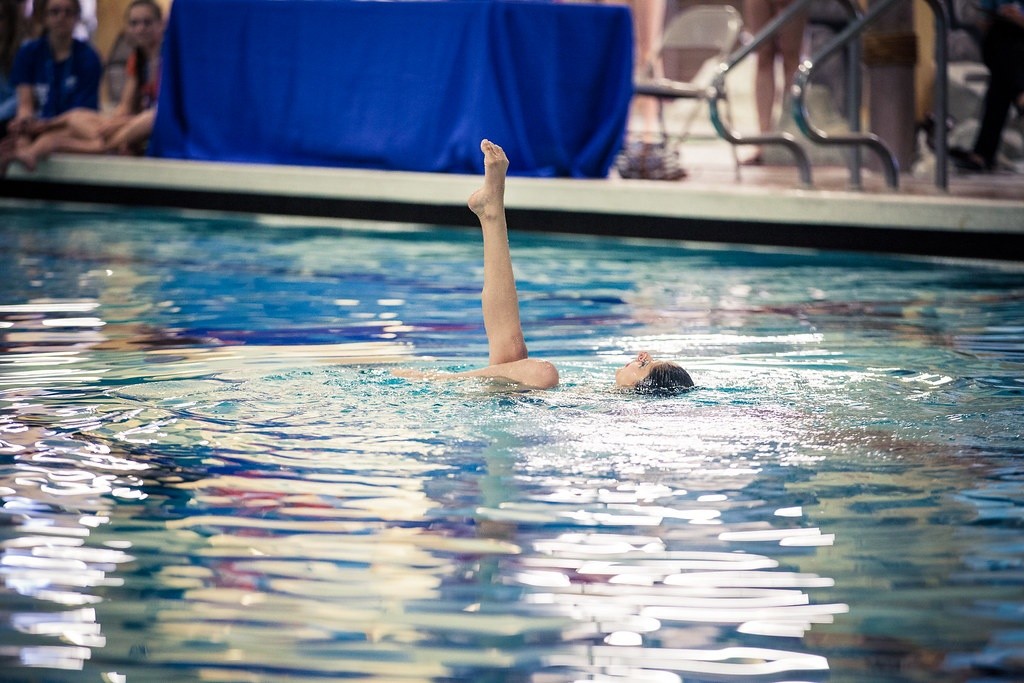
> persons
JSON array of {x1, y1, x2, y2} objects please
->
[
  {"x1": 390, "y1": 138, "x2": 694, "y2": 397},
  {"x1": 599, "y1": 1, "x2": 667, "y2": 147},
  {"x1": 943, "y1": 0, "x2": 1024, "y2": 172},
  {"x1": 0, "y1": 0, "x2": 166, "y2": 172},
  {"x1": 741, "y1": 0, "x2": 871, "y2": 166}
]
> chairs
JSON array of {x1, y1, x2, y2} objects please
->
[{"x1": 634, "y1": 5, "x2": 742, "y2": 171}]
